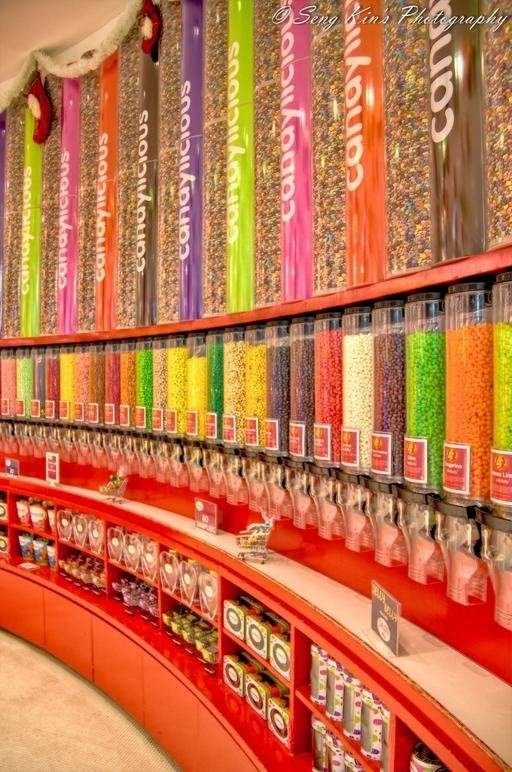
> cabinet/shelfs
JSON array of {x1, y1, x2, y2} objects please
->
[{"x1": 0, "y1": 246, "x2": 511, "y2": 771}]
